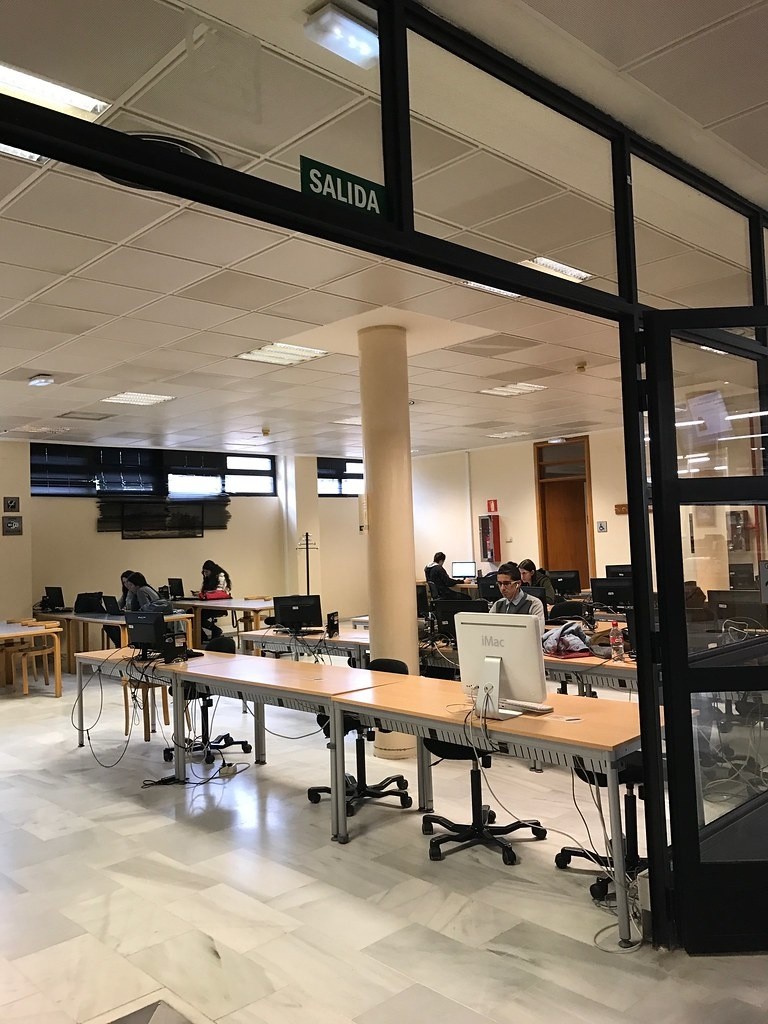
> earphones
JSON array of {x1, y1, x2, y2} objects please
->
[{"x1": 516, "y1": 584, "x2": 519, "y2": 588}]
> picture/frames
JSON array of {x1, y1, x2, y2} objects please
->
[
  {"x1": 2, "y1": 516, "x2": 22, "y2": 535},
  {"x1": 4, "y1": 497, "x2": 19, "y2": 512}
]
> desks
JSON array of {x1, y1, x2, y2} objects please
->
[{"x1": 0, "y1": 580, "x2": 704, "y2": 948}]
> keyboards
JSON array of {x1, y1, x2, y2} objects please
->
[
  {"x1": 467, "y1": 695, "x2": 553, "y2": 713},
  {"x1": 273, "y1": 629, "x2": 324, "y2": 633}
]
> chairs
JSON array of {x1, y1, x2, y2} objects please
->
[
  {"x1": 684, "y1": 586, "x2": 761, "y2": 771},
  {"x1": 163, "y1": 637, "x2": 252, "y2": 763},
  {"x1": 308, "y1": 658, "x2": 411, "y2": 817},
  {"x1": 427, "y1": 581, "x2": 440, "y2": 599},
  {"x1": 237, "y1": 596, "x2": 273, "y2": 649},
  {"x1": 11, "y1": 621, "x2": 60, "y2": 695},
  {"x1": 550, "y1": 601, "x2": 594, "y2": 621},
  {"x1": 122, "y1": 678, "x2": 191, "y2": 742},
  {"x1": 555, "y1": 750, "x2": 648, "y2": 902},
  {"x1": 419, "y1": 738, "x2": 548, "y2": 865},
  {"x1": 0, "y1": 617, "x2": 38, "y2": 688}
]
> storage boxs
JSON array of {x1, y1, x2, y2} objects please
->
[{"x1": 637, "y1": 869, "x2": 653, "y2": 943}]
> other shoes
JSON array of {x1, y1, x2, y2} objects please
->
[{"x1": 212, "y1": 628, "x2": 223, "y2": 638}]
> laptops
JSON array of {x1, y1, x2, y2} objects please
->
[{"x1": 102, "y1": 596, "x2": 125, "y2": 615}]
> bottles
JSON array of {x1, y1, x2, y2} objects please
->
[{"x1": 610, "y1": 621, "x2": 624, "y2": 660}]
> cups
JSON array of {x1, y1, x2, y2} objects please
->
[{"x1": 478, "y1": 569, "x2": 482, "y2": 577}]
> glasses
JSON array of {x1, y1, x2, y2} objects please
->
[{"x1": 496, "y1": 581, "x2": 517, "y2": 587}]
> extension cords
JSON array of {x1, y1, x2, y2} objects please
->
[{"x1": 219, "y1": 763, "x2": 237, "y2": 774}]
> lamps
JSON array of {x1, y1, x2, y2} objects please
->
[
  {"x1": 26, "y1": 375, "x2": 56, "y2": 387},
  {"x1": 301, "y1": 3, "x2": 379, "y2": 72}
]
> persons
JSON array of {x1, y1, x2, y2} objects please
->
[
  {"x1": 103, "y1": 570, "x2": 162, "y2": 648},
  {"x1": 488, "y1": 559, "x2": 555, "y2": 636},
  {"x1": 185, "y1": 560, "x2": 231, "y2": 645},
  {"x1": 424, "y1": 552, "x2": 472, "y2": 600}
]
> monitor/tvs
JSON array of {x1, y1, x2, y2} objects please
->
[
  {"x1": 168, "y1": 578, "x2": 184, "y2": 596},
  {"x1": 686, "y1": 391, "x2": 734, "y2": 439},
  {"x1": 452, "y1": 562, "x2": 476, "y2": 578},
  {"x1": 454, "y1": 612, "x2": 547, "y2": 721},
  {"x1": 124, "y1": 612, "x2": 165, "y2": 661},
  {"x1": 707, "y1": 590, "x2": 761, "y2": 621},
  {"x1": 590, "y1": 578, "x2": 633, "y2": 613},
  {"x1": 273, "y1": 594, "x2": 323, "y2": 636},
  {"x1": 45, "y1": 587, "x2": 65, "y2": 607},
  {"x1": 521, "y1": 587, "x2": 547, "y2": 620},
  {"x1": 548, "y1": 570, "x2": 581, "y2": 596},
  {"x1": 605, "y1": 565, "x2": 632, "y2": 578},
  {"x1": 430, "y1": 601, "x2": 489, "y2": 648}
]
